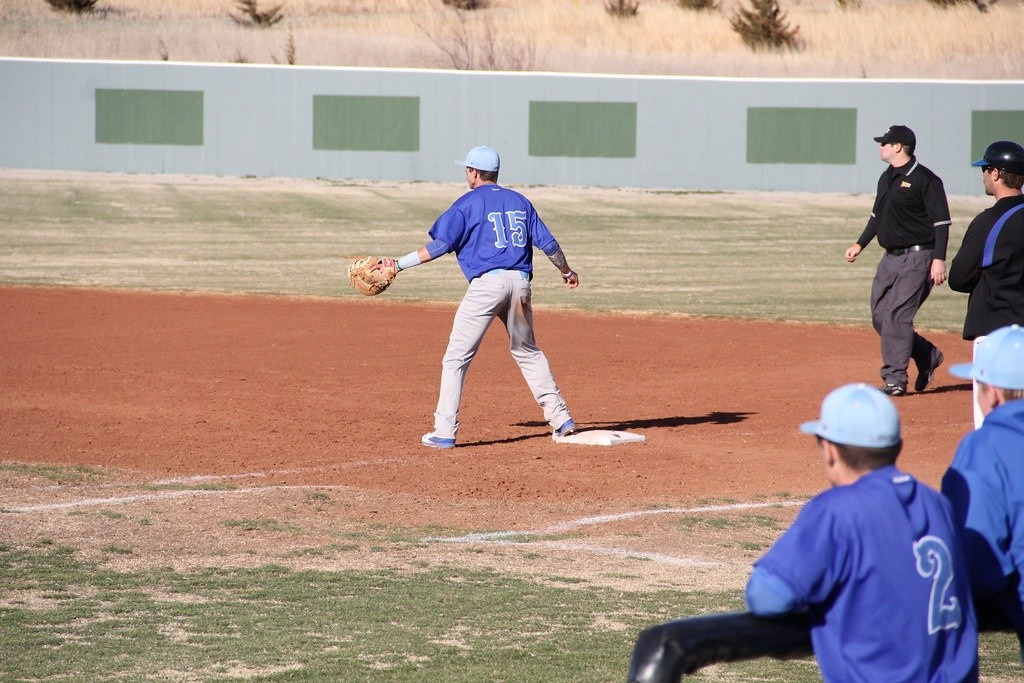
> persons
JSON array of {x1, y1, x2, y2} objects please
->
[
  {"x1": 941, "y1": 324, "x2": 1024, "y2": 659},
  {"x1": 745, "y1": 383, "x2": 978, "y2": 683},
  {"x1": 845, "y1": 125, "x2": 952, "y2": 395},
  {"x1": 348, "y1": 145, "x2": 579, "y2": 449},
  {"x1": 948, "y1": 140, "x2": 1024, "y2": 430}
]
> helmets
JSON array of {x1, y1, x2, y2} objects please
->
[{"x1": 972, "y1": 141, "x2": 1024, "y2": 175}]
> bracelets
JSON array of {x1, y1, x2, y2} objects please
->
[
  {"x1": 854, "y1": 244, "x2": 862, "y2": 250},
  {"x1": 562, "y1": 271, "x2": 572, "y2": 278}
]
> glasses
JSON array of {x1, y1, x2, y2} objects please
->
[{"x1": 982, "y1": 165, "x2": 1000, "y2": 172}]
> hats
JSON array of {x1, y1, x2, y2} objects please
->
[
  {"x1": 949, "y1": 324, "x2": 1024, "y2": 388},
  {"x1": 800, "y1": 382, "x2": 901, "y2": 447},
  {"x1": 873, "y1": 124, "x2": 916, "y2": 146},
  {"x1": 454, "y1": 145, "x2": 500, "y2": 171}
]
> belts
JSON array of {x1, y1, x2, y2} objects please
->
[
  {"x1": 888, "y1": 243, "x2": 936, "y2": 256},
  {"x1": 487, "y1": 268, "x2": 528, "y2": 277}
]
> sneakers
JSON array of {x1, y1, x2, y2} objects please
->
[
  {"x1": 914, "y1": 350, "x2": 944, "y2": 393},
  {"x1": 877, "y1": 382, "x2": 908, "y2": 395},
  {"x1": 421, "y1": 433, "x2": 455, "y2": 449},
  {"x1": 561, "y1": 418, "x2": 576, "y2": 435}
]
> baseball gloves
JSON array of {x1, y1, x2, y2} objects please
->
[{"x1": 347, "y1": 256, "x2": 399, "y2": 297}]
